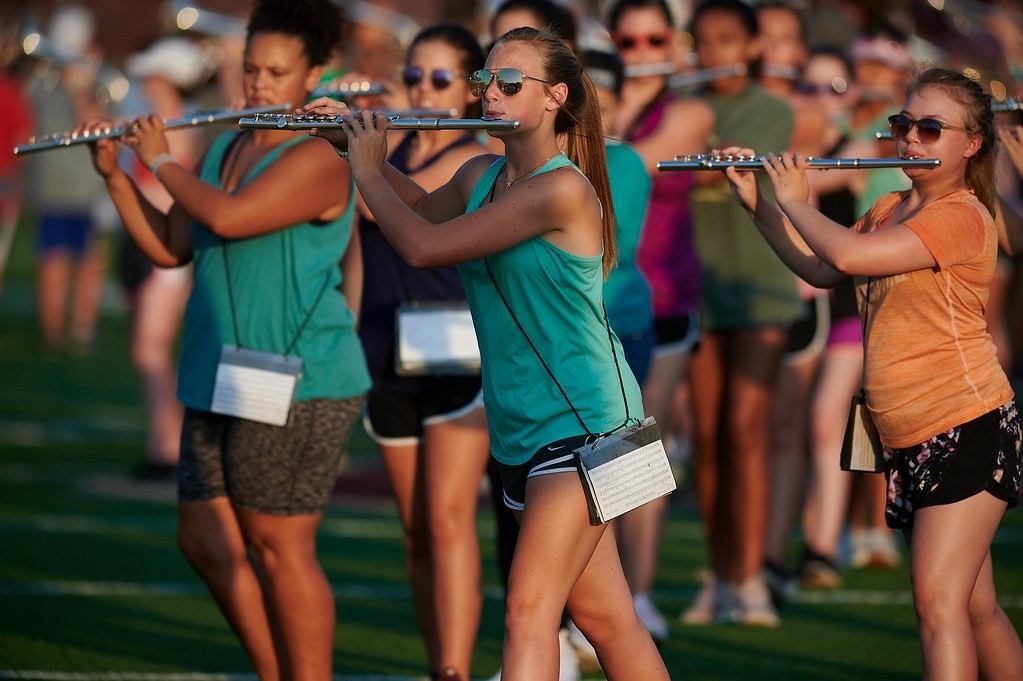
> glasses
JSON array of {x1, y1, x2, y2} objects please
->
[
  {"x1": 470, "y1": 68, "x2": 559, "y2": 97},
  {"x1": 798, "y1": 81, "x2": 850, "y2": 98},
  {"x1": 889, "y1": 115, "x2": 976, "y2": 144},
  {"x1": 614, "y1": 31, "x2": 670, "y2": 51},
  {"x1": 403, "y1": 68, "x2": 471, "y2": 91}
]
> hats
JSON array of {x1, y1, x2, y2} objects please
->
[
  {"x1": 127, "y1": 38, "x2": 208, "y2": 85},
  {"x1": 49, "y1": 8, "x2": 92, "y2": 62}
]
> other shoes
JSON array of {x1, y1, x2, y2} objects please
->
[
  {"x1": 726, "y1": 578, "x2": 780, "y2": 626},
  {"x1": 633, "y1": 594, "x2": 667, "y2": 637},
  {"x1": 490, "y1": 628, "x2": 580, "y2": 681},
  {"x1": 853, "y1": 531, "x2": 899, "y2": 570},
  {"x1": 795, "y1": 546, "x2": 840, "y2": 603},
  {"x1": 681, "y1": 582, "x2": 717, "y2": 625},
  {"x1": 567, "y1": 623, "x2": 604, "y2": 669}
]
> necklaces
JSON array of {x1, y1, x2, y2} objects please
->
[{"x1": 505, "y1": 152, "x2": 563, "y2": 189}]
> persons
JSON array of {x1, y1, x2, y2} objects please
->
[
  {"x1": 294, "y1": 25, "x2": 677, "y2": 680},
  {"x1": 710, "y1": 66, "x2": 1023, "y2": 680},
  {"x1": 0, "y1": 1, "x2": 1022, "y2": 680},
  {"x1": 63, "y1": 0, "x2": 374, "y2": 681}
]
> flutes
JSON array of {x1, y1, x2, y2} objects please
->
[
  {"x1": 238, "y1": 113, "x2": 522, "y2": 134},
  {"x1": 14, "y1": 101, "x2": 290, "y2": 156},
  {"x1": 609, "y1": 60, "x2": 1016, "y2": 113},
  {"x1": 310, "y1": 84, "x2": 383, "y2": 99},
  {"x1": 657, "y1": 153, "x2": 941, "y2": 172}
]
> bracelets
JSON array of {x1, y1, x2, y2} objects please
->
[
  {"x1": 150, "y1": 155, "x2": 177, "y2": 175},
  {"x1": 333, "y1": 146, "x2": 350, "y2": 161}
]
